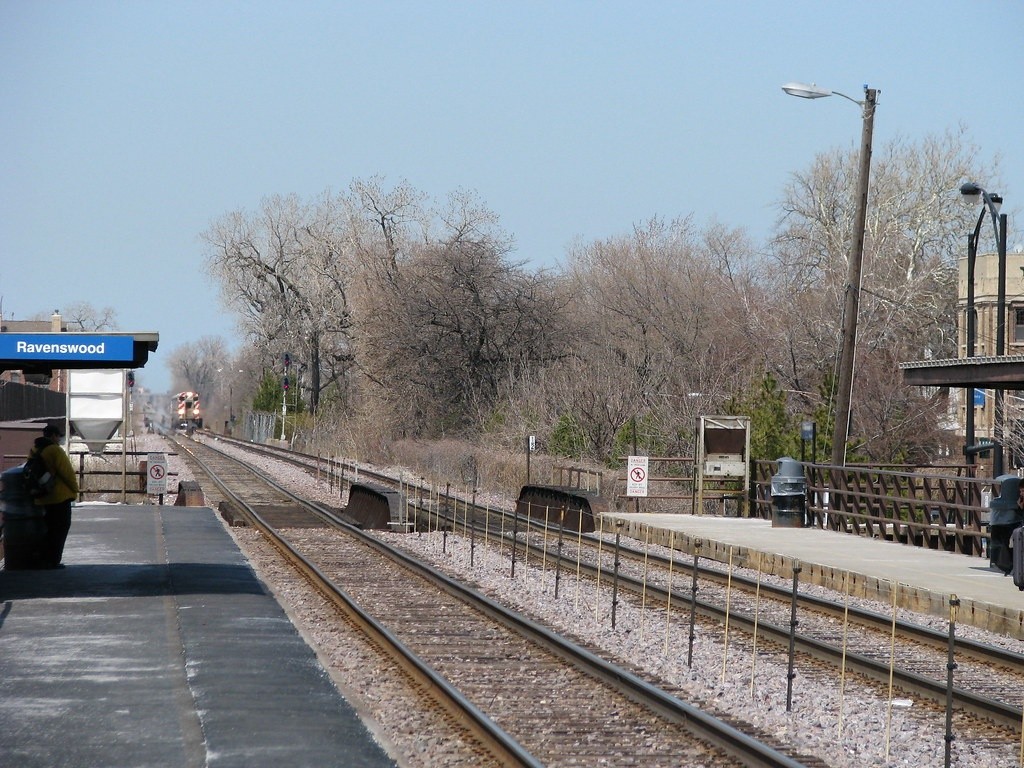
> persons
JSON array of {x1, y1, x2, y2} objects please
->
[{"x1": 25, "y1": 424, "x2": 80, "y2": 570}]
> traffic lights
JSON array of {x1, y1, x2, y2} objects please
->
[
  {"x1": 285, "y1": 354, "x2": 290, "y2": 367},
  {"x1": 127, "y1": 371, "x2": 135, "y2": 388},
  {"x1": 285, "y1": 377, "x2": 289, "y2": 390}
]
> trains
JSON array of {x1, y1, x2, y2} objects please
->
[{"x1": 170, "y1": 391, "x2": 202, "y2": 430}]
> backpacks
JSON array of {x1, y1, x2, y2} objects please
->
[{"x1": 21, "y1": 447, "x2": 55, "y2": 499}]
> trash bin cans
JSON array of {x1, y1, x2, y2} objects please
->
[
  {"x1": 990, "y1": 475, "x2": 1021, "y2": 573},
  {"x1": 771, "y1": 457, "x2": 806, "y2": 528}
]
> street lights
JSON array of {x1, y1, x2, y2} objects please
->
[
  {"x1": 963, "y1": 191, "x2": 1005, "y2": 555},
  {"x1": 780, "y1": 82, "x2": 884, "y2": 530},
  {"x1": 958, "y1": 182, "x2": 1006, "y2": 479}
]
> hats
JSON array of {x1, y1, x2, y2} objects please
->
[{"x1": 42, "y1": 424, "x2": 64, "y2": 437}]
